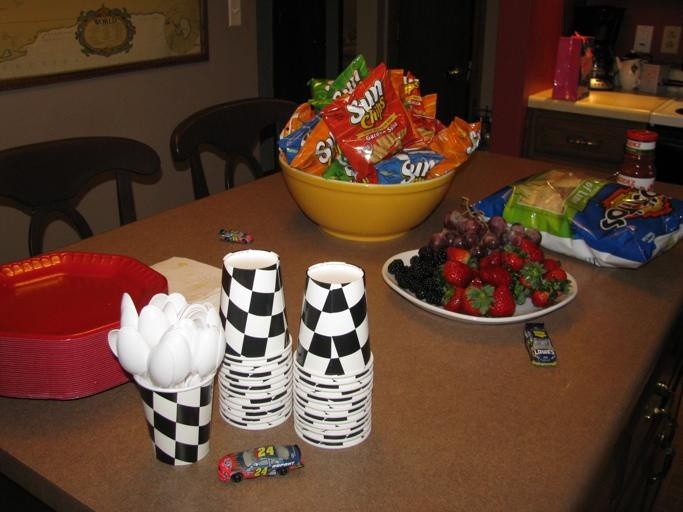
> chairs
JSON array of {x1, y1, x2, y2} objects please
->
[
  {"x1": 166, "y1": 100, "x2": 295, "y2": 203},
  {"x1": 1, "y1": 133, "x2": 164, "y2": 260}
]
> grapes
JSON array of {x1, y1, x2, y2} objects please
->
[{"x1": 429, "y1": 197, "x2": 542, "y2": 261}]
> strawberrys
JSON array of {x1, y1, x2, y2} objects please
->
[{"x1": 442, "y1": 240, "x2": 571, "y2": 318}]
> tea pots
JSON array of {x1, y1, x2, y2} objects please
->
[{"x1": 615, "y1": 56, "x2": 648, "y2": 92}]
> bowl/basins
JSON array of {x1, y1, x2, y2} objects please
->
[{"x1": 277, "y1": 150, "x2": 455, "y2": 243}]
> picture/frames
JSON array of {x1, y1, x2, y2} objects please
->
[{"x1": 0, "y1": 0, "x2": 211, "y2": 92}]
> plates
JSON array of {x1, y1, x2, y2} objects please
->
[
  {"x1": 381, "y1": 248, "x2": 580, "y2": 325},
  {"x1": 0, "y1": 250, "x2": 171, "y2": 399}
]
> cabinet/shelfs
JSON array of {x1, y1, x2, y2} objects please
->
[{"x1": 521, "y1": 108, "x2": 648, "y2": 173}]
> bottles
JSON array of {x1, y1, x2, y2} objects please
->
[{"x1": 616, "y1": 129, "x2": 658, "y2": 192}]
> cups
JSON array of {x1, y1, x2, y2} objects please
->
[
  {"x1": 132, "y1": 372, "x2": 214, "y2": 466},
  {"x1": 218, "y1": 250, "x2": 374, "y2": 448}
]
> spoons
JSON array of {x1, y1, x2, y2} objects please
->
[{"x1": 106, "y1": 287, "x2": 228, "y2": 387}]
